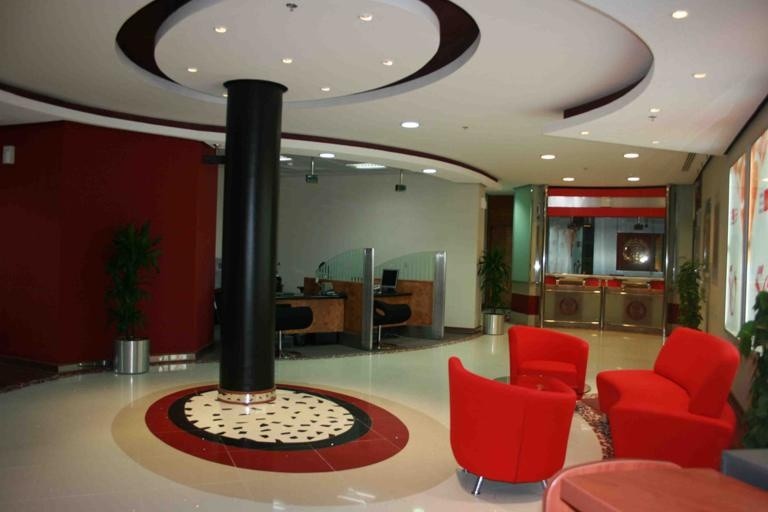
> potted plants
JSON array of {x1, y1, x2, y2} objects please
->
[
  {"x1": 476, "y1": 240, "x2": 510, "y2": 336},
  {"x1": 103, "y1": 218, "x2": 165, "y2": 376}
]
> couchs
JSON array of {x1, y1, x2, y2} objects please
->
[
  {"x1": 542, "y1": 461, "x2": 741, "y2": 510},
  {"x1": 507, "y1": 325, "x2": 591, "y2": 406},
  {"x1": 598, "y1": 324, "x2": 742, "y2": 474},
  {"x1": 447, "y1": 356, "x2": 578, "y2": 494}
]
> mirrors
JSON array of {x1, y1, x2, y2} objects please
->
[{"x1": 547, "y1": 212, "x2": 664, "y2": 279}]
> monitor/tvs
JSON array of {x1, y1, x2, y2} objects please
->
[{"x1": 381, "y1": 269, "x2": 399, "y2": 292}]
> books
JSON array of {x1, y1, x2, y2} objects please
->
[
  {"x1": 274, "y1": 292, "x2": 305, "y2": 299},
  {"x1": 319, "y1": 290, "x2": 339, "y2": 296}
]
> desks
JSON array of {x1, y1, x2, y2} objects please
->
[
  {"x1": 276, "y1": 290, "x2": 345, "y2": 345},
  {"x1": 373, "y1": 290, "x2": 413, "y2": 339},
  {"x1": 490, "y1": 373, "x2": 592, "y2": 394}
]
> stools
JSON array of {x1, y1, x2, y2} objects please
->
[
  {"x1": 374, "y1": 300, "x2": 410, "y2": 352},
  {"x1": 275, "y1": 302, "x2": 312, "y2": 361}
]
